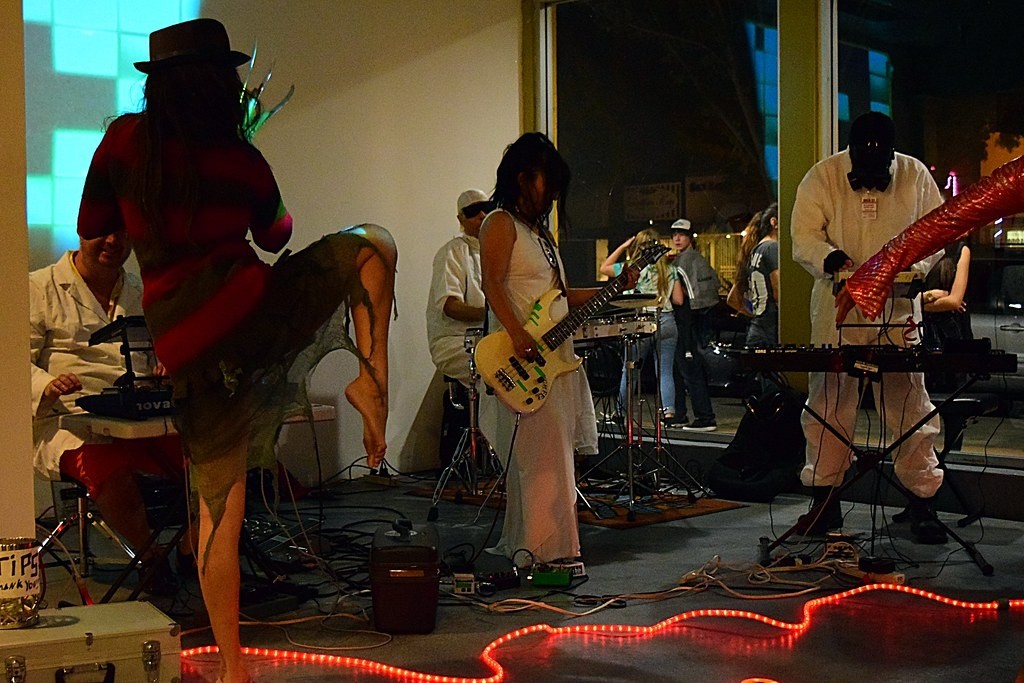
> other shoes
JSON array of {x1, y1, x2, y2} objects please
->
[
  {"x1": 141, "y1": 553, "x2": 180, "y2": 596},
  {"x1": 181, "y1": 553, "x2": 200, "y2": 582}
]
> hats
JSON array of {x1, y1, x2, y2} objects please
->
[
  {"x1": 669, "y1": 218, "x2": 694, "y2": 236},
  {"x1": 132, "y1": 18, "x2": 252, "y2": 76},
  {"x1": 630, "y1": 229, "x2": 657, "y2": 251}
]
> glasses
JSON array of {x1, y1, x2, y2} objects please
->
[{"x1": 461, "y1": 200, "x2": 498, "y2": 219}]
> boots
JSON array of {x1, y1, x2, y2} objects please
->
[
  {"x1": 904, "y1": 497, "x2": 950, "y2": 545},
  {"x1": 798, "y1": 485, "x2": 844, "y2": 530}
]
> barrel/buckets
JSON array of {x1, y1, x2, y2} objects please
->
[{"x1": 0, "y1": 537, "x2": 40, "y2": 631}]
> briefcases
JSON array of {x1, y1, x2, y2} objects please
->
[{"x1": 1, "y1": 601, "x2": 183, "y2": 683}]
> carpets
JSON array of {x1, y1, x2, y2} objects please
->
[{"x1": 406, "y1": 481, "x2": 748, "y2": 528}]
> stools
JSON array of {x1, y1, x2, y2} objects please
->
[
  {"x1": 429, "y1": 376, "x2": 507, "y2": 514},
  {"x1": 37, "y1": 474, "x2": 147, "y2": 578}
]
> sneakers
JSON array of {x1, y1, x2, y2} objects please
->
[
  {"x1": 682, "y1": 418, "x2": 718, "y2": 432},
  {"x1": 595, "y1": 415, "x2": 624, "y2": 428},
  {"x1": 658, "y1": 415, "x2": 690, "y2": 429}
]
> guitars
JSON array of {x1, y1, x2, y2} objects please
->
[{"x1": 475, "y1": 240, "x2": 672, "y2": 414}]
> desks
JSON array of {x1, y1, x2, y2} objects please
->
[{"x1": 58, "y1": 401, "x2": 336, "y2": 608}]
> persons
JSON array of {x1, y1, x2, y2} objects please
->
[
  {"x1": 598, "y1": 229, "x2": 685, "y2": 426},
  {"x1": 917, "y1": 238, "x2": 971, "y2": 452},
  {"x1": 426, "y1": 189, "x2": 494, "y2": 390},
  {"x1": 27, "y1": 230, "x2": 202, "y2": 587},
  {"x1": 72, "y1": 19, "x2": 398, "y2": 683},
  {"x1": 723, "y1": 201, "x2": 779, "y2": 347},
  {"x1": 660, "y1": 218, "x2": 725, "y2": 433},
  {"x1": 479, "y1": 131, "x2": 640, "y2": 571},
  {"x1": 787, "y1": 110, "x2": 952, "y2": 547}
]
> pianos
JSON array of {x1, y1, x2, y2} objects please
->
[
  {"x1": 74, "y1": 313, "x2": 175, "y2": 421},
  {"x1": 752, "y1": 341, "x2": 1019, "y2": 575},
  {"x1": 463, "y1": 312, "x2": 662, "y2": 522}
]
